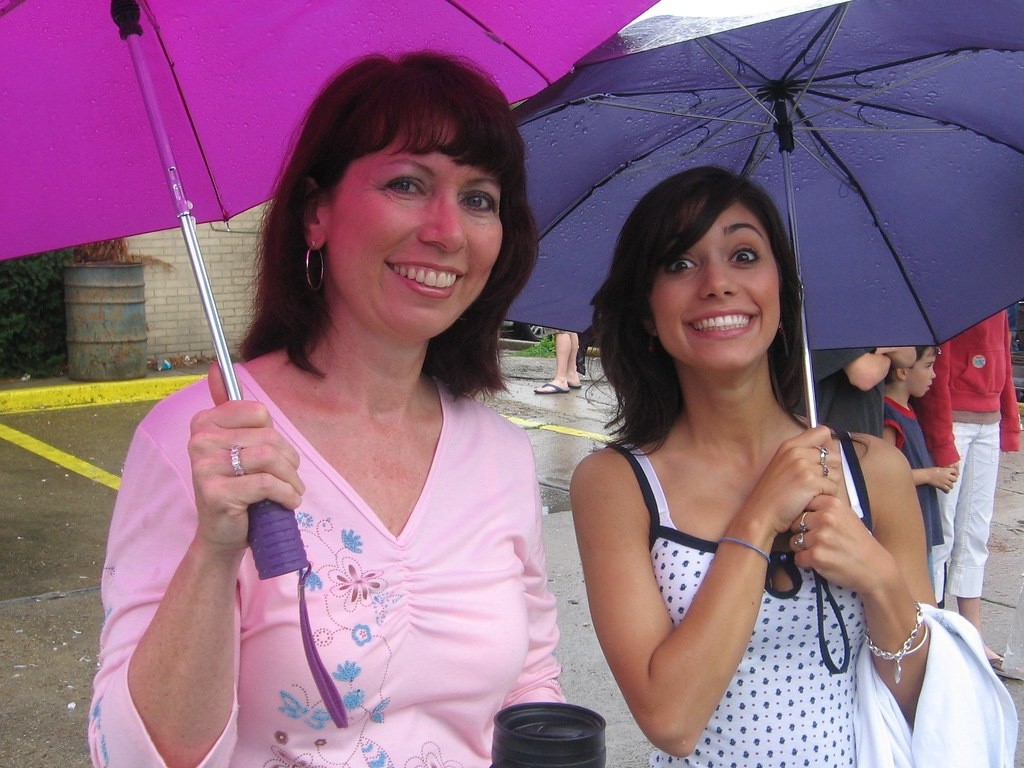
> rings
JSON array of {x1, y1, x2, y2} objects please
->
[
  {"x1": 815, "y1": 445, "x2": 829, "y2": 464},
  {"x1": 823, "y1": 464, "x2": 829, "y2": 477},
  {"x1": 794, "y1": 533, "x2": 806, "y2": 550},
  {"x1": 231, "y1": 445, "x2": 244, "y2": 476},
  {"x1": 799, "y1": 512, "x2": 808, "y2": 532}
]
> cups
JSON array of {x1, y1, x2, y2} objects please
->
[{"x1": 491, "y1": 702, "x2": 606, "y2": 768}]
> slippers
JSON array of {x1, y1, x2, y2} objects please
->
[
  {"x1": 534, "y1": 383, "x2": 570, "y2": 394},
  {"x1": 567, "y1": 381, "x2": 582, "y2": 389}
]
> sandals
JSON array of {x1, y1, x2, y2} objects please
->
[{"x1": 988, "y1": 654, "x2": 1024, "y2": 680}]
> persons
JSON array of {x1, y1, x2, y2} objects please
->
[
  {"x1": 909, "y1": 308, "x2": 1020, "y2": 679},
  {"x1": 811, "y1": 345, "x2": 916, "y2": 439},
  {"x1": 534, "y1": 331, "x2": 582, "y2": 394},
  {"x1": 569, "y1": 161, "x2": 938, "y2": 768},
  {"x1": 881, "y1": 346, "x2": 960, "y2": 592},
  {"x1": 86, "y1": 49, "x2": 566, "y2": 767}
]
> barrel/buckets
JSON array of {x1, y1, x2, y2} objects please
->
[{"x1": 64, "y1": 262, "x2": 148, "y2": 381}]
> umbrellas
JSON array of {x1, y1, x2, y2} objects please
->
[
  {"x1": 506, "y1": 0, "x2": 1024, "y2": 433},
  {"x1": 0, "y1": 0, "x2": 663, "y2": 579}
]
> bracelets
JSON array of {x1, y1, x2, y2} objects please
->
[
  {"x1": 865, "y1": 600, "x2": 928, "y2": 683},
  {"x1": 718, "y1": 537, "x2": 770, "y2": 563}
]
